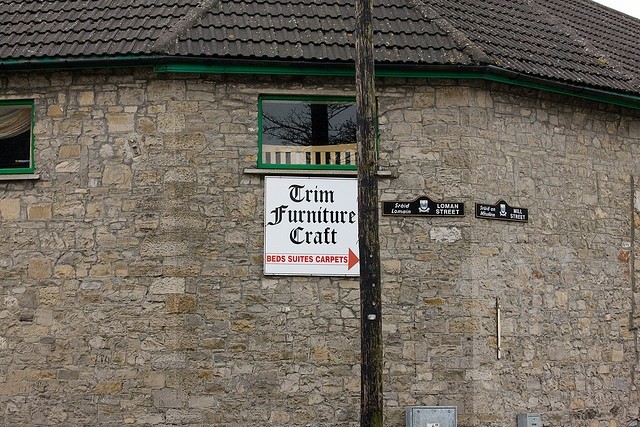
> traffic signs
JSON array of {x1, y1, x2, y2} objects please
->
[{"x1": 263, "y1": 174, "x2": 360, "y2": 277}]
